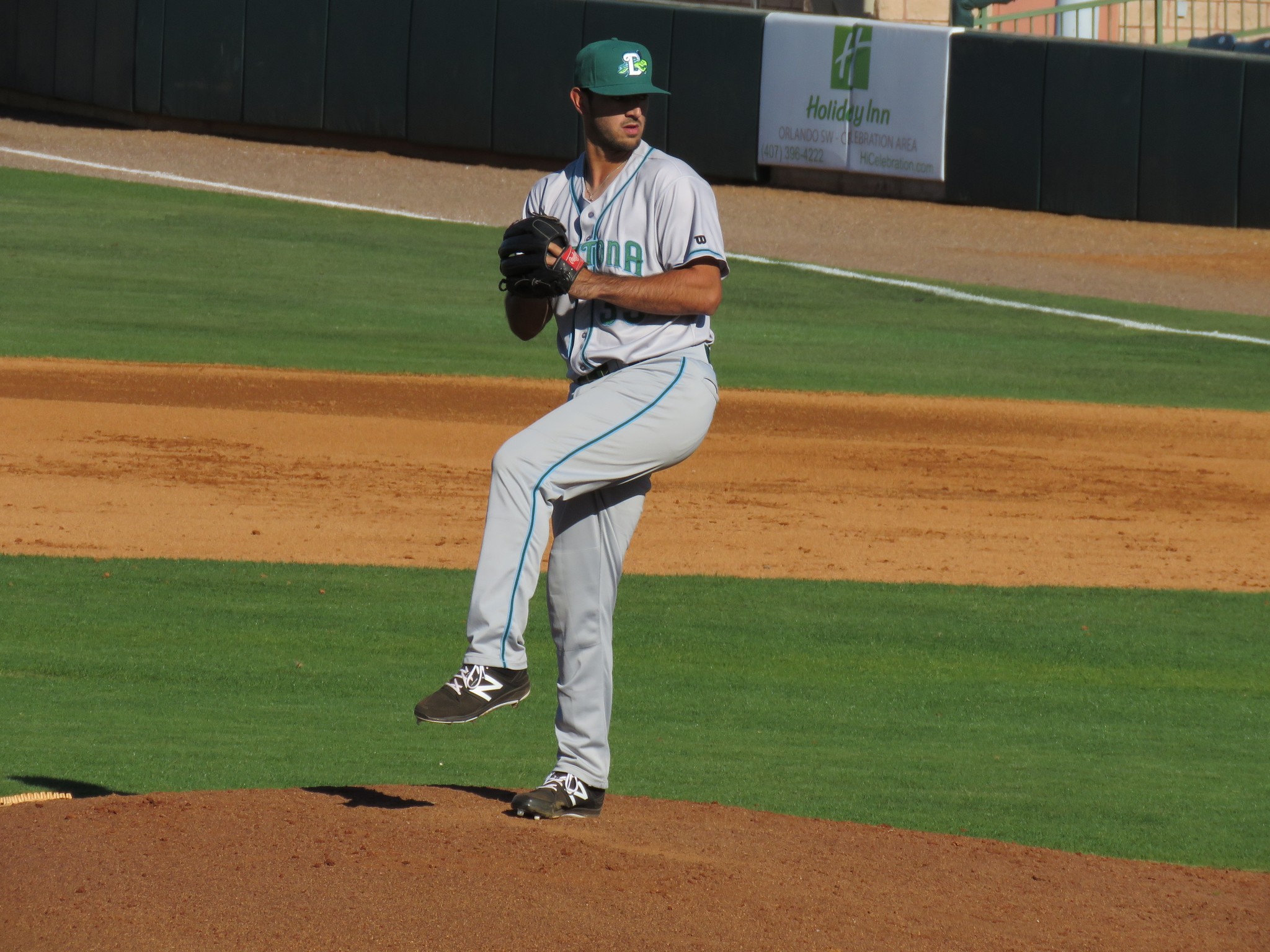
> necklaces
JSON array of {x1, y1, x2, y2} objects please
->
[{"x1": 585, "y1": 158, "x2": 629, "y2": 201}]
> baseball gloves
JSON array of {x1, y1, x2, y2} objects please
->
[{"x1": 497, "y1": 214, "x2": 588, "y2": 299}]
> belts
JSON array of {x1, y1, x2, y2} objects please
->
[{"x1": 574, "y1": 358, "x2": 653, "y2": 386}]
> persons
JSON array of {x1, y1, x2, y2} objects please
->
[{"x1": 414, "y1": 38, "x2": 725, "y2": 820}]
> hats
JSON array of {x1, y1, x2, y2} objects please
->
[{"x1": 575, "y1": 38, "x2": 671, "y2": 95}]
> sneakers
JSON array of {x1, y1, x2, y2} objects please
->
[
  {"x1": 510, "y1": 771, "x2": 605, "y2": 820},
  {"x1": 414, "y1": 663, "x2": 531, "y2": 726}
]
